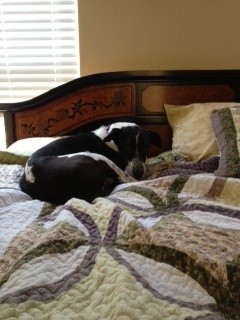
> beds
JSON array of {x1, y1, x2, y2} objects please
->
[{"x1": 0, "y1": 70, "x2": 240, "y2": 320}]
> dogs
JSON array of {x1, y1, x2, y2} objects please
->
[{"x1": 19, "y1": 119, "x2": 163, "y2": 205}]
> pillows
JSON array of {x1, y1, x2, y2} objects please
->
[
  {"x1": 6, "y1": 136, "x2": 67, "y2": 158},
  {"x1": 211, "y1": 106, "x2": 240, "y2": 177},
  {"x1": 163, "y1": 102, "x2": 240, "y2": 163}
]
